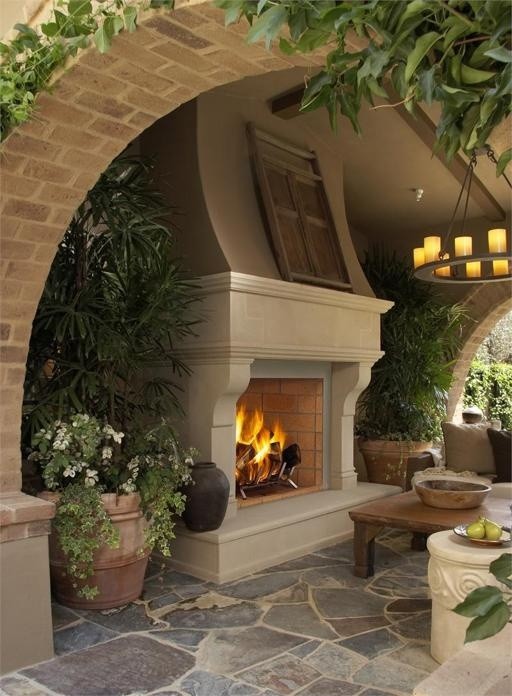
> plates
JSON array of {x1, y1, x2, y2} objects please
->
[{"x1": 453, "y1": 523, "x2": 511, "y2": 547}]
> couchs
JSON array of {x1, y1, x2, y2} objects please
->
[{"x1": 404, "y1": 448, "x2": 512, "y2": 552}]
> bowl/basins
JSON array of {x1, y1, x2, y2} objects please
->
[{"x1": 415, "y1": 478, "x2": 492, "y2": 509}]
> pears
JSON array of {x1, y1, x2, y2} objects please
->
[{"x1": 466, "y1": 515, "x2": 502, "y2": 541}]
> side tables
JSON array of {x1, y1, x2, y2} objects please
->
[{"x1": 426, "y1": 525, "x2": 512, "y2": 665}]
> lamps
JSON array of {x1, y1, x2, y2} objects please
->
[{"x1": 410, "y1": 137, "x2": 512, "y2": 285}]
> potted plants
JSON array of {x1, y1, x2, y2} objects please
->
[
  {"x1": 353, "y1": 287, "x2": 482, "y2": 493},
  {"x1": 22, "y1": 151, "x2": 217, "y2": 610}
]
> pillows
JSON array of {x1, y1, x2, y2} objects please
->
[
  {"x1": 441, "y1": 420, "x2": 502, "y2": 474},
  {"x1": 486, "y1": 426, "x2": 512, "y2": 483}
]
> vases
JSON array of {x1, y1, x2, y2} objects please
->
[{"x1": 179, "y1": 462, "x2": 230, "y2": 533}]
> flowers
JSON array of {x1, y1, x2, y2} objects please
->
[{"x1": 28, "y1": 403, "x2": 198, "y2": 596}]
